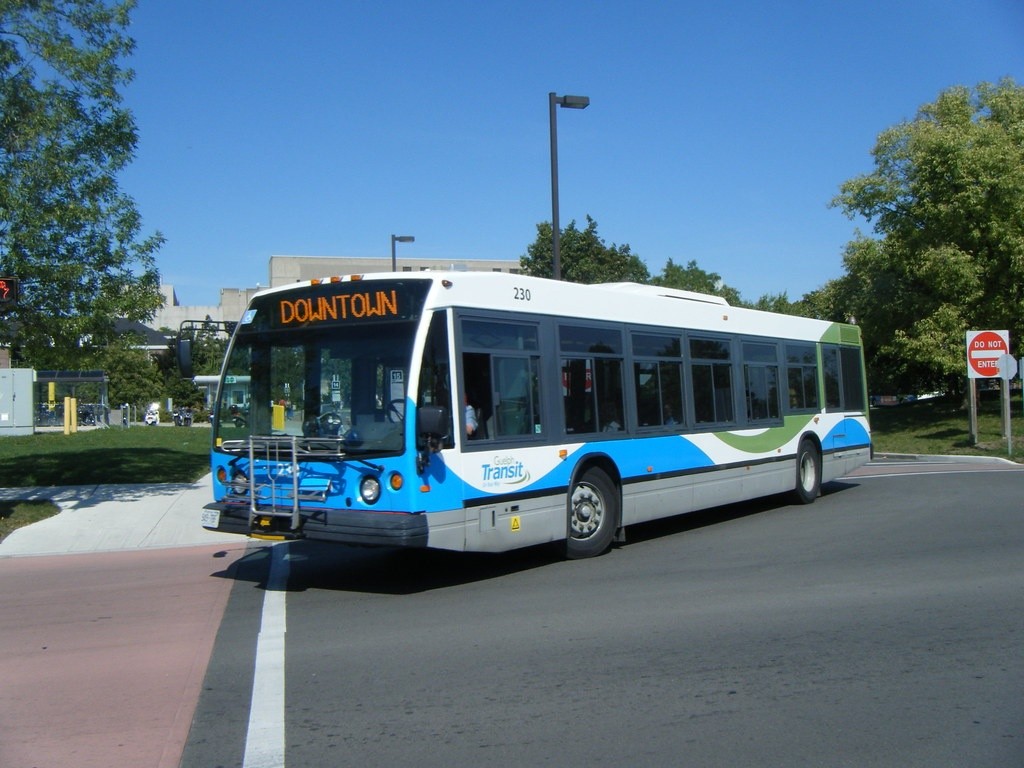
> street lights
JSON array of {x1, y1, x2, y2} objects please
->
[
  {"x1": 546, "y1": 90, "x2": 590, "y2": 280},
  {"x1": 390, "y1": 235, "x2": 417, "y2": 269}
]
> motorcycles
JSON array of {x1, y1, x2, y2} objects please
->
[
  {"x1": 228, "y1": 403, "x2": 250, "y2": 430},
  {"x1": 207, "y1": 409, "x2": 222, "y2": 428},
  {"x1": 144, "y1": 409, "x2": 160, "y2": 427}
]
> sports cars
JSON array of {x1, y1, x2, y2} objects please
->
[{"x1": 172, "y1": 408, "x2": 195, "y2": 428}]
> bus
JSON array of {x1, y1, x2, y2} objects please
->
[{"x1": 176, "y1": 268, "x2": 875, "y2": 559}]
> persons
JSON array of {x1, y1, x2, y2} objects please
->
[
  {"x1": 433, "y1": 385, "x2": 478, "y2": 449},
  {"x1": 584, "y1": 392, "x2": 744, "y2": 431},
  {"x1": 285, "y1": 398, "x2": 292, "y2": 421},
  {"x1": 279, "y1": 397, "x2": 286, "y2": 408}
]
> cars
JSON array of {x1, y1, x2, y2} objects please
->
[{"x1": 301, "y1": 408, "x2": 351, "y2": 440}]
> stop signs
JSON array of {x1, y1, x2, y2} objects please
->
[{"x1": 966, "y1": 332, "x2": 1011, "y2": 376}]
